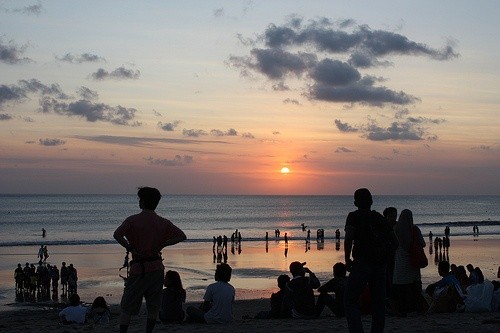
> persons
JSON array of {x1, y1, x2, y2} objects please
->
[
  {"x1": 159, "y1": 270, "x2": 186, "y2": 323},
  {"x1": 242, "y1": 261, "x2": 321, "y2": 320},
  {"x1": 113, "y1": 185, "x2": 188, "y2": 333},
  {"x1": 335, "y1": 229, "x2": 341, "y2": 242},
  {"x1": 186, "y1": 263, "x2": 236, "y2": 325},
  {"x1": 14, "y1": 245, "x2": 78, "y2": 296},
  {"x1": 160, "y1": 253, "x2": 164, "y2": 262},
  {"x1": 90, "y1": 296, "x2": 112, "y2": 325},
  {"x1": 266, "y1": 222, "x2": 324, "y2": 245},
  {"x1": 119, "y1": 251, "x2": 130, "y2": 273},
  {"x1": 383, "y1": 207, "x2": 500, "y2": 318},
  {"x1": 58, "y1": 294, "x2": 90, "y2": 325},
  {"x1": 41, "y1": 228, "x2": 46, "y2": 237},
  {"x1": 315, "y1": 262, "x2": 349, "y2": 317},
  {"x1": 213, "y1": 228, "x2": 242, "y2": 250},
  {"x1": 344, "y1": 187, "x2": 399, "y2": 333}
]
